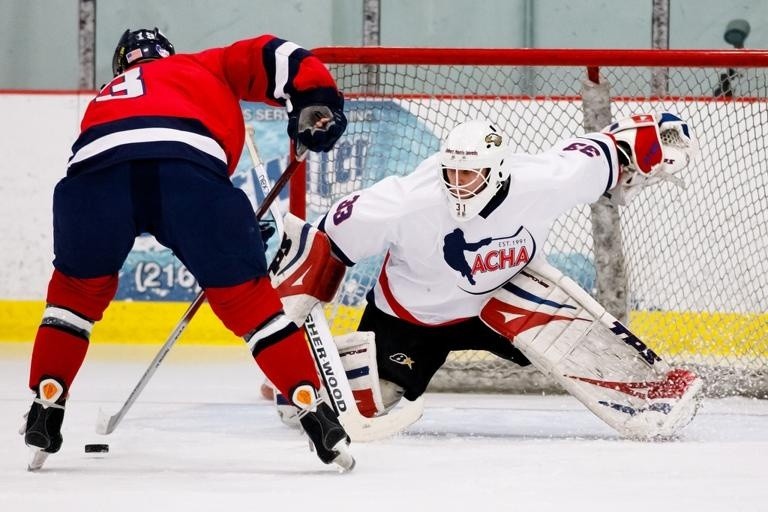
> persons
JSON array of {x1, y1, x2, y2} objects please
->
[
  {"x1": 22, "y1": 27, "x2": 351, "y2": 463},
  {"x1": 260, "y1": 112, "x2": 698, "y2": 440}
]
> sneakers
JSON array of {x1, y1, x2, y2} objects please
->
[
  {"x1": 289, "y1": 383, "x2": 350, "y2": 462},
  {"x1": 25, "y1": 373, "x2": 66, "y2": 452}
]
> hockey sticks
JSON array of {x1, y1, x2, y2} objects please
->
[
  {"x1": 242, "y1": 122, "x2": 424, "y2": 442},
  {"x1": 68, "y1": 110, "x2": 332, "y2": 436}
]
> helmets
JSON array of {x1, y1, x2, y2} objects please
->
[
  {"x1": 439, "y1": 120, "x2": 510, "y2": 223},
  {"x1": 112, "y1": 27, "x2": 174, "y2": 76}
]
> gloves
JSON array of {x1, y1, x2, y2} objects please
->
[{"x1": 287, "y1": 87, "x2": 347, "y2": 152}]
